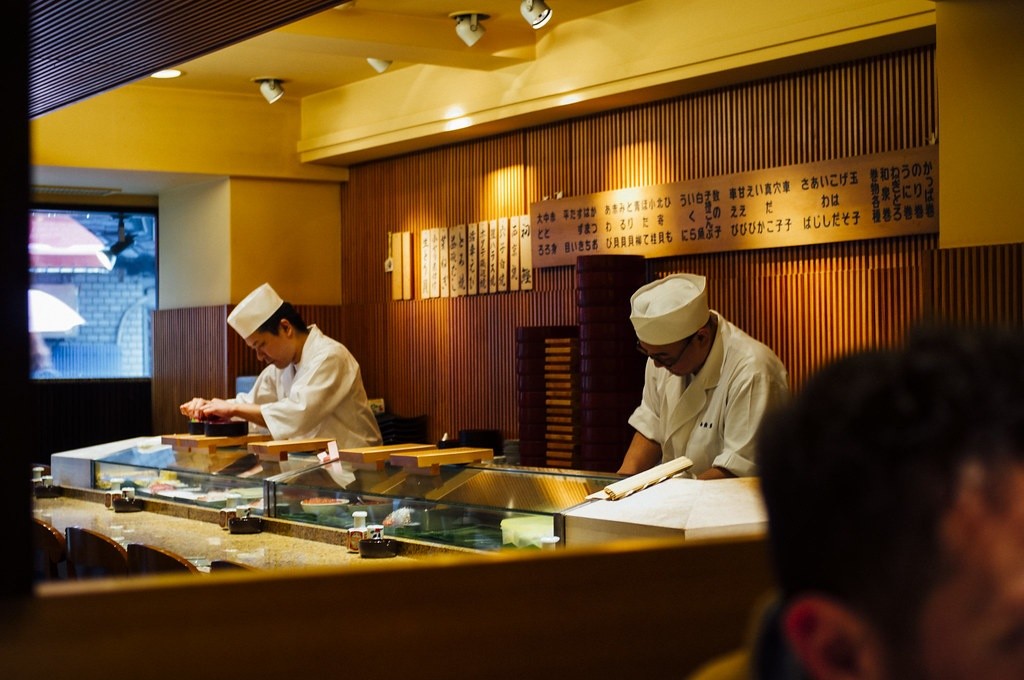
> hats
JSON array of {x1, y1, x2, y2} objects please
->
[
  {"x1": 630, "y1": 273, "x2": 710, "y2": 345},
  {"x1": 226, "y1": 283, "x2": 283, "y2": 339}
]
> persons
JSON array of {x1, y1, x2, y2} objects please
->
[
  {"x1": 738, "y1": 324, "x2": 1024, "y2": 680},
  {"x1": 30, "y1": 333, "x2": 62, "y2": 379},
  {"x1": 180, "y1": 282, "x2": 382, "y2": 449},
  {"x1": 616, "y1": 273, "x2": 789, "y2": 479}
]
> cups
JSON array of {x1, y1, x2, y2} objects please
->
[
  {"x1": 236, "y1": 506, "x2": 251, "y2": 516},
  {"x1": 122, "y1": 489, "x2": 134, "y2": 499},
  {"x1": 367, "y1": 526, "x2": 384, "y2": 539},
  {"x1": 42, "y1": 478, "x2": 52, "y2": 486}
]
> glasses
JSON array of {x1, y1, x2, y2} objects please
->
[{"x1": 637, "y1": 331, "x2": 699, "y2": 367}]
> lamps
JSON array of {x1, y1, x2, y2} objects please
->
[
  {"x1": 455, "y1": 14, "x2": 482, "y2": 47},
  {"x1": 96, "y1": 213, "x2": 136, "y2": 271},
  {"x1": 259, "y1": 79, "x2": 283, "y2": 104},
  {"x1": 520, "y1": 0, "x2": 553, "y2": 30},
  {"x1": 366, "y1": 58, "x2": 393, "y2": 75}
]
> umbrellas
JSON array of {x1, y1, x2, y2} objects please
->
[{"x1": 28, "y1": 289, "x2": 87, "y2": 332}]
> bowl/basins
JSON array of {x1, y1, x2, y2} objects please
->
[
  {"x1": 188, "y1": 422, "x2": 205, "y2": 435},
  {"x1": 302, "y1": 499, "x2": 348, "y2": 516},
  {"x1": 205, "y1": 421, "x2": 249, "y2": 436}
]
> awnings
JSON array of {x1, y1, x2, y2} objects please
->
[{"x1": 30, "y1": 213, "x2": 112, "y2": 270}]
